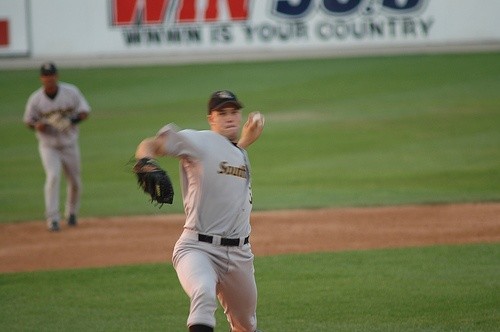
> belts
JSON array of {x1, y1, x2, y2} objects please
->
[{"x1": 198, "y1": 232, "x2": 250, "y2": 247}]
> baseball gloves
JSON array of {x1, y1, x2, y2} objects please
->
[
  {"x1": 133, "y1": 158, "x2": 174, "y2": 209},
  {"x1": 32, "y1": 110, "x2": 70, "y2": 140}
]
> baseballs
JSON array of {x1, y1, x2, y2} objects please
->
[{"x1": 253, "y1": 114, "x2": 265, "y2": 126}]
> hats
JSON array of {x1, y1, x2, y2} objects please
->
[
  {"x1": 40, "y1": 61, "x2": 57, "y2": 75},
  {"x1": 209, "y1": 90, "x2": 240, "y2": 109}
]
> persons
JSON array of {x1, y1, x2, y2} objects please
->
[
  {"x1": 24, "y1": 63, "x2": 91, "y2": 232},
  {"x1": 134, "y1": 90, "x2": 265, "y2": 332}
]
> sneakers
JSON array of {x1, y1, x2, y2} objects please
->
[{"x1": 46, "y1": 214, "x2": 77, "y2": 232}]
